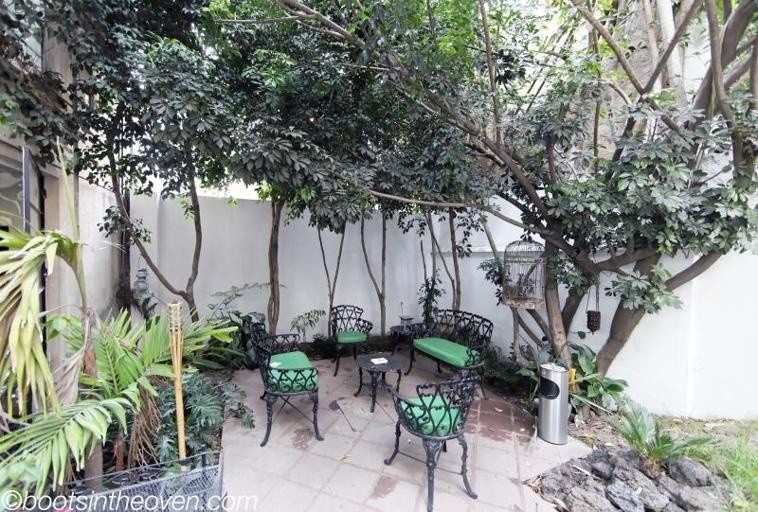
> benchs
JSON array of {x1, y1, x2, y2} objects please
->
[
  {"x1": 404, "y1": 308, "x2": 493, "y2": 400},
  {"x1": 249, "y1": 322, "x2": 324, "y2": 447}
]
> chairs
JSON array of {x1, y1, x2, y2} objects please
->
[
  {"x1": 384, "y1": 376, "x2": 482, "y2": 512},
  {"x1": 330, "y1": 304, "x2": 373, "y2": 377}
]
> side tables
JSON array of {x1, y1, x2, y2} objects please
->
[{"x1": 390, "y1": 325, "x2": 416, "y2": 361}]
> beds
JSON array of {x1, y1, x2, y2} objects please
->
[{"x1": 354, "y1": 353, "x2": 401, "y2": 413}]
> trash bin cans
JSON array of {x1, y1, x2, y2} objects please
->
[{"x1": 537, "y1": 361, "x2": 569, "y2": 445}]
